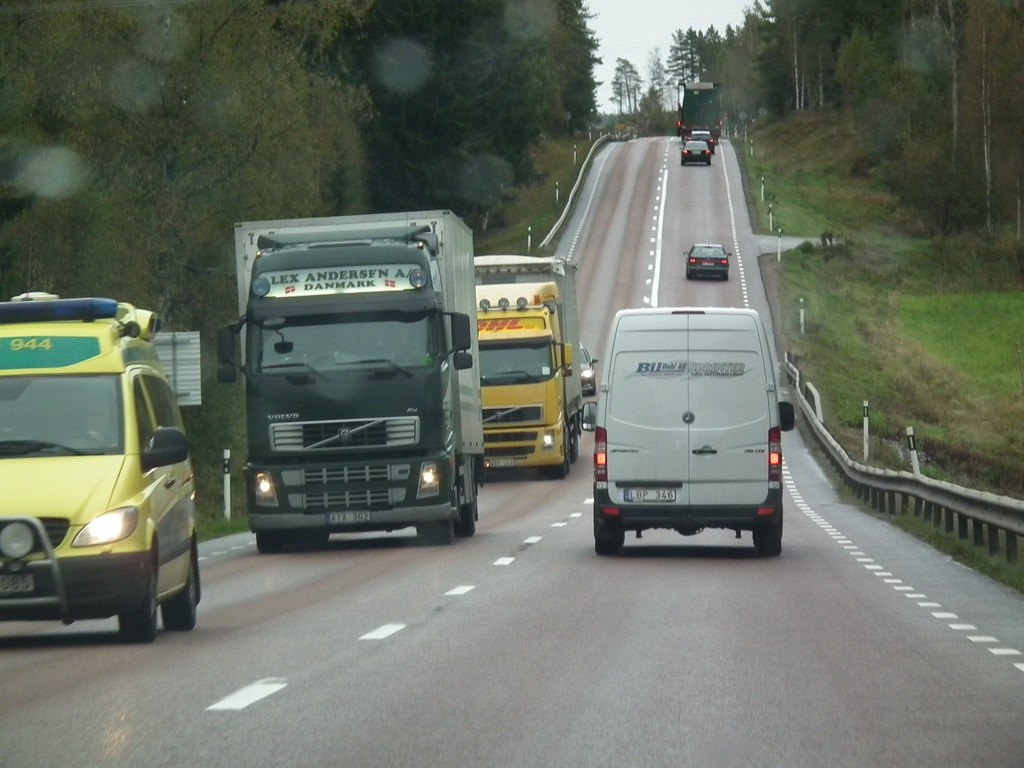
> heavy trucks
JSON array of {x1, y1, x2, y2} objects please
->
[
  {"x1": 217, "y1": 209, "x2": 488, "y2": 554},
  {"x1": 466, "y1": 255, "x2": 585, "y2": 479},
  {"x1": 677, "y1": 82, "x2": 724, "y2": 145}
]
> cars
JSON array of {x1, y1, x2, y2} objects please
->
[
  {"x1": 689, "y1": 131, "x2": 715, "y2": 154},
  {"x1": 579, "y1": 342, "x2": 599, "y2": 396},
  {"x1": 684, "y1": 244, "x2": 732, "y2": 281},
  {"x1": 680, "y1": 141, "x2": 711, "y2": 167}
]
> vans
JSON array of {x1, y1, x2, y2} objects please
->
[
  {"x1": 0, "y1": 294, "x2": 203, "y2": 644},
  {"x1": 578, "y1": 307, "x2": 798, "y2": 558}
]
